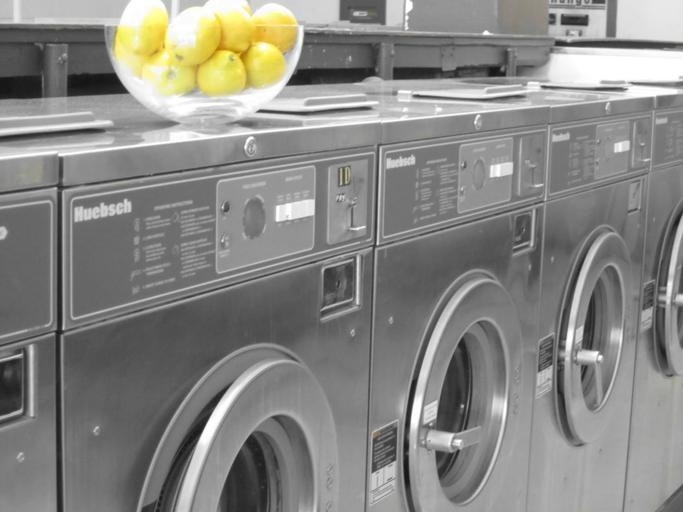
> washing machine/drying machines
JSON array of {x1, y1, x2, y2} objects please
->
[{"x1": 1, "y1": 1, "x2": 683, "y2": 510}]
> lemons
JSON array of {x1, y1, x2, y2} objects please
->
[{"x1": 113, "y1": 0, "x2": 298, "y2": 97}]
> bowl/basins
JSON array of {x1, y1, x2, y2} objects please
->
[{"x1": 102, "y1": 18, "x2": 305, "y2": 127}]
